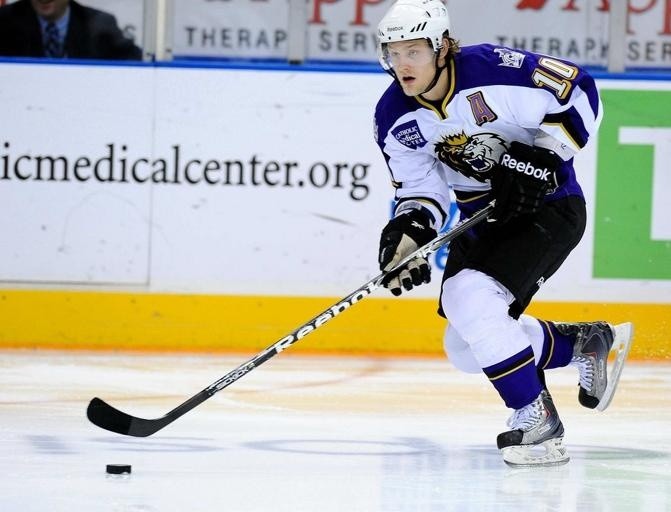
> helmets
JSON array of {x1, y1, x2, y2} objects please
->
[{"x1": 376, "y1": 0, "x2": 449, "y2": 70}]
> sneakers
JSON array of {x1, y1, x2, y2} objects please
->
[
  {"x1": 497, "y1": 390, "x2": 564, "y2": 449},
  {"x1": 572, "y1": 322, "x2": 614, "y2": 408}
]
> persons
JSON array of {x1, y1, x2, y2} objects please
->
[
  {"x1": 373, "y1": 1, "x2": 615, "y2": 452},
  {"x1": 1, "y1": 0, "x2": 144, "y2": 61}
]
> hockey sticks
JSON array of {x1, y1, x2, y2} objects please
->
[{"x1": 86, "y1": 198, "x2": 496, "y2": 437}]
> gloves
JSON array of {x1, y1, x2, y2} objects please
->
[
  {"x1": 379, "y1": 208, "x2": 437, "y2": 295},
  {"x1": 494, "y1": 141, "x2": 554, "y2": 224}
]
